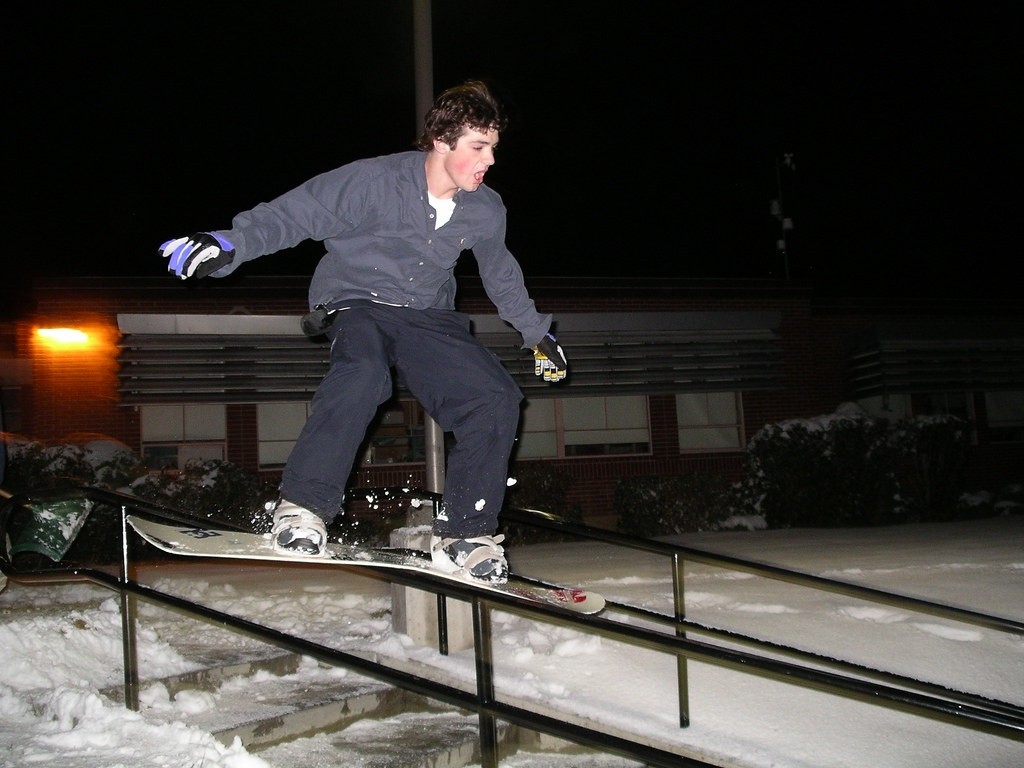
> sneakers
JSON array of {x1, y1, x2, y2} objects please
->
[
  {"x1": 429, "y1": 532, "x2": 508, "y2": 585},
  {"x1": 272, "y1": 496, "x2": 327, "y2": 554}
]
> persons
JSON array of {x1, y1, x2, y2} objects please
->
[{"x1": 158, "y1": 84, "x2": 567, "y2": 586}]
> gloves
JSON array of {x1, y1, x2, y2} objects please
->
[
  {"x1": 157, "y1": 231, "x2": 235, "y2": 280},
  {"x1": 530, "y1": 333, "x2": 568, "y2": 382}
]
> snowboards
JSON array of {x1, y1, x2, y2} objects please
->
[{"x1": 126, "y1": 514, "x2": 606, "y2": 616}]
